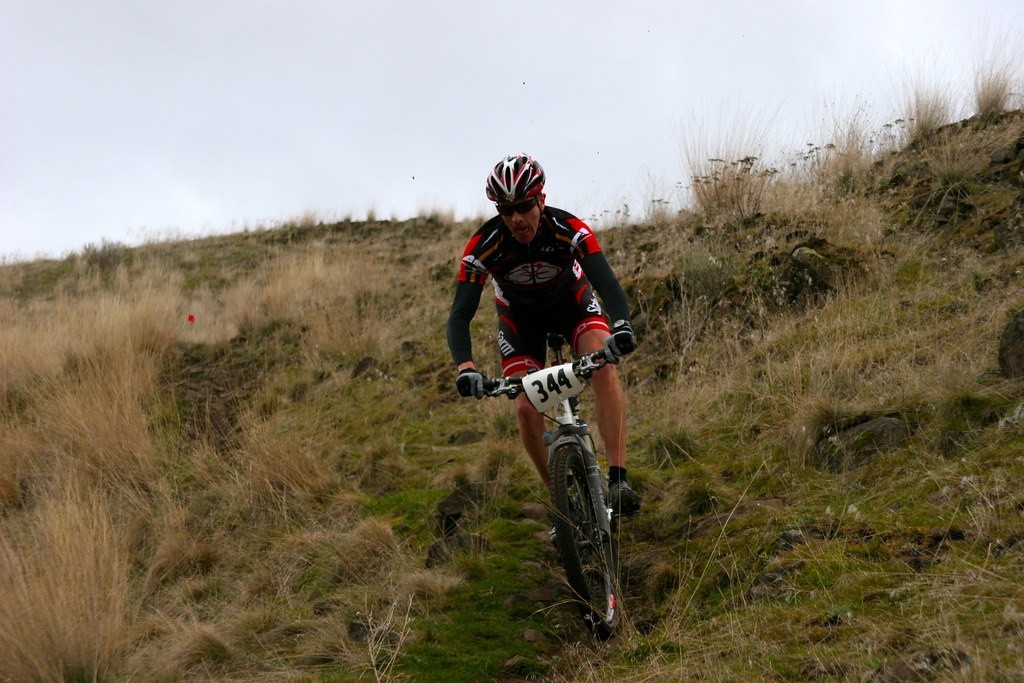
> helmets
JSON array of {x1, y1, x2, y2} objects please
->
[{"x1": 486, "y1": 153, "x2": 545, "y2": 204}]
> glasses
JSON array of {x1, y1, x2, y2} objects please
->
[{"x1": 495, "y1": 193, "x2": 542, "y2": 217}]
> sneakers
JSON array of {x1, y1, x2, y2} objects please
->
[
  {"x1": 608, "y1": 483, "x2": 641, "y2": 516},
  {"x1": 549, "y1": 507, "x2": 580, "y2": 548}
]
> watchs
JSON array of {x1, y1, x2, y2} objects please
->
[{"x1": 613, "y1": 320, "x2": 630, "y2": 328}]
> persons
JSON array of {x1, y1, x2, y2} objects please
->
[{"x1": 446, "y1": 154, "x2": 639, "y2": 547}]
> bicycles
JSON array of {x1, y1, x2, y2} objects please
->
[{"x1": 478, "y1": 327, "x2": 627, "y2": 641}]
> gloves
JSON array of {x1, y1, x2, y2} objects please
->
[
  {"x1": 603, "y1": 325, "x2": 638, "y2": 365},
  {"x1": 456, "y1": 369, "x2": 488, "y2": 399}
]
